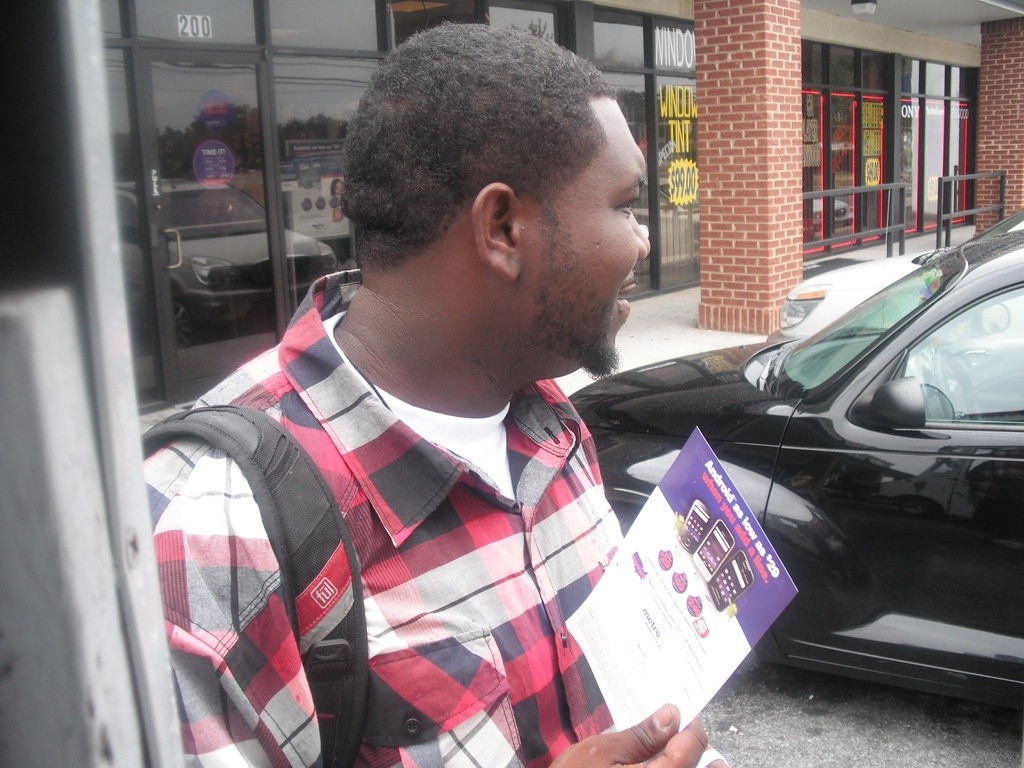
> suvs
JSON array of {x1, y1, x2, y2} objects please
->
[{"x1": 112, "y1": 177, "x2": 340, "y2": 351}]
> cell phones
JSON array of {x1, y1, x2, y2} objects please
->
[{"x1": 678, "y1": 500, "x2": 755, "y2": 612}]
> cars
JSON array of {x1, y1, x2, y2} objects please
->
[
  {"x1": 781, "y1": 243, "x2": 955, "y2": 343},
  {"x1": 566, "y1": 228, "x2": 1024, "y2": 714}
]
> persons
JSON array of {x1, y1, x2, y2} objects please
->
[{"x1": 143, "y1": 23, "x2": 733, "y2": 767}]
